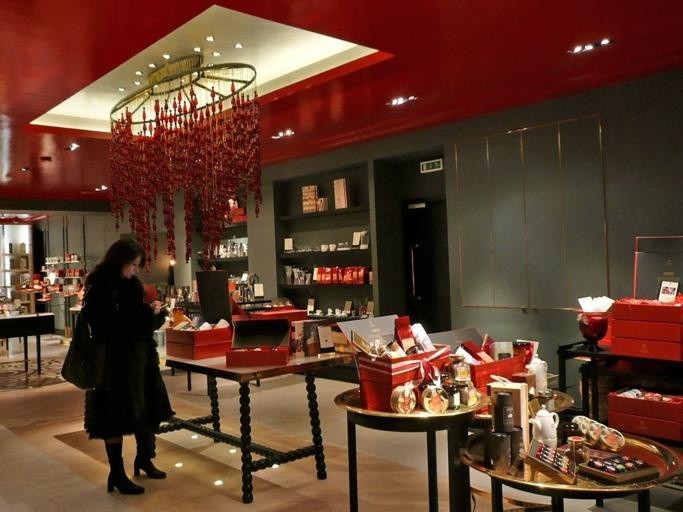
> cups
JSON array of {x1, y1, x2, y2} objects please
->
[
  {"x1": 316, "y1": 310, "x2": 322, "y2": 313},
  {"x1": 321, "y1": 244, "x2": 336, "y2": 252}
]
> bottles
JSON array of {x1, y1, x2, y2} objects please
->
[
  {"x1": 417, "y1": 355, "x2": 472, "y2": 411},
  {"x1": 561, "y1": 421, "x2": 590, "y2": 465}
]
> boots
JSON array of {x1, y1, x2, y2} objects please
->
[
  {"x1": 107, "y1": 457, "x2": 145, "y2": 494},
  {"x1": 133, "y1": 454, "x2": 166, "y2": 480}
]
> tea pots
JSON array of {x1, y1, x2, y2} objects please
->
[
  {"x1": 529, "y1": 405, "x2": 559, "y2": 442},
  {"x1": 525, "y1": 354, "x2": 548, "y2": 391}
]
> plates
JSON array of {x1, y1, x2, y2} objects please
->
[
  {"x1": 327, "y1": 314, "x2": 334, "y2": 316},
  {"x1": 314, "y1": 313, "x2": 320, "y2": 315}
]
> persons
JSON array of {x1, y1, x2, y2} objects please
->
[{"x1": 82, "y1": 237, "x2": 176, "y2": 495}]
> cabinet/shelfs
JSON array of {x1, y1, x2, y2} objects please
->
[
  {"x1": 271, "y1": 160, "x2": 373, "y2": 321},
  {"x1": 188, "y1": 185, "x2": 248, "y2": 262}
]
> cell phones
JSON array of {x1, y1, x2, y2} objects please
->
[{"x1": 159, "y1": 303, "x2": 168, "y2": 309}]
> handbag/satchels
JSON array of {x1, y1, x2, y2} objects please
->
[{"x1": 62, "y1": 308, "x2": 99, "y2": 388}]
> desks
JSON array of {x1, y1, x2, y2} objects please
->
[
  {"x1": 333, "y1": 383, "x2": 490, "y2": 512},
  {"x1": 461, "y1": 424, "x2": 680, "y2": 511},
  {"x1": 460, "y1": 388, "x2": 576, "y2": 511},
  {"x1": 156, "y1": 344, "x2": 355, "y2": 504}
]
